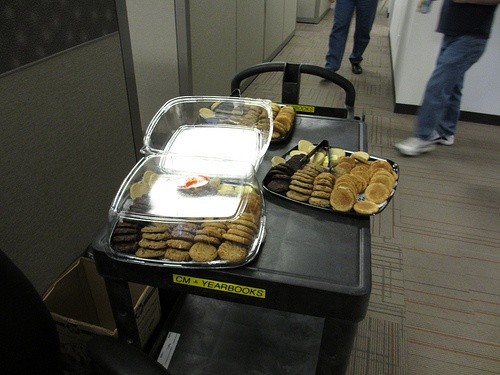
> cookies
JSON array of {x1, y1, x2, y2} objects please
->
[
  {"x1": 109, "y1": 170, "x2": 263, "y2": 261},
  {"x1": 271, "y1": 140, "x2": 398, "y2": 214},
  {"x1": 228, "y1": 102, "x2": 296, "y2": 139}
]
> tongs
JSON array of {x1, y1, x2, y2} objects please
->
[{"x1": 299, "y1": 140, "x2": 332, "y2": 172}]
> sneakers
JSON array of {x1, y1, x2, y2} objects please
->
[
  {"x1": 393, "y1": 136, "x2": 437, "y2": 156},
  {"x1": 426, "y1": 129, "x2": 455, "y2": 145}
]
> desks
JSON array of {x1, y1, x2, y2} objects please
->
[{"x1": 94, "y1": 115, "x2": 371, "y2": 375}]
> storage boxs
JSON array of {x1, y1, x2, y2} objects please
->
[{"x1": 42, "y1": 256, "x2": 166, "y2": 362}]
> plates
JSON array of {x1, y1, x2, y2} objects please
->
[
  {"x1": 104, "y1": 194, "x2": 266, "y2": 270},
  {"x1": 267, "y1": 108, "x2": 297, "y2": 148},
  {"x1": 261, "y1": 143, "x2": 400, "y2": 216}
]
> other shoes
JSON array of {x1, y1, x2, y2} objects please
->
[
  {"x1": 320, "y1": 64, "x2": 335, "y2": 81},
  {"x1": 352, "y1": 62, "x2": 362, "y2": 74}
]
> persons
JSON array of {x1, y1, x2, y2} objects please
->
[
  {"x1": 392, "y1": 0, "x2": 500, "y2": 158},
  {"x1": 318, "y1": 0, "x2": 378, "y2": 82}
]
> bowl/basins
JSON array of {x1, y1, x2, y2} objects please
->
[{"x1": 142, "y1": 95, "x2": 274, "y2": 166}]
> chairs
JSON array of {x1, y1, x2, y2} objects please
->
[{"x1": 232, "y1": 61, "x2": 358, "y2": 118}]
